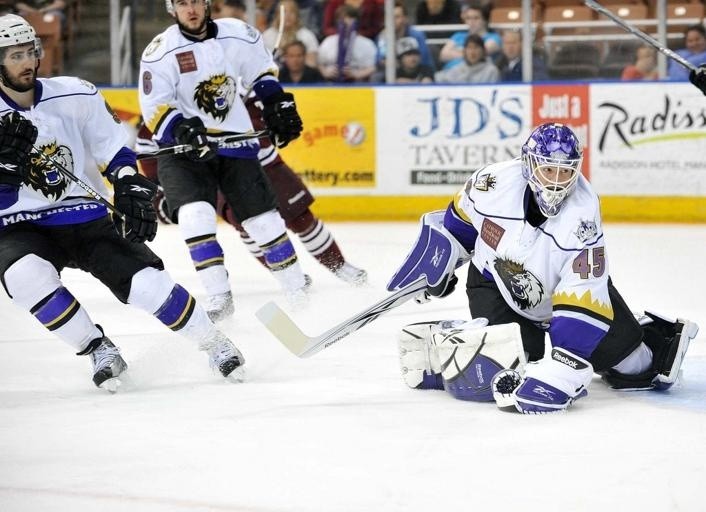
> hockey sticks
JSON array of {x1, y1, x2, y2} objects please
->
[{"x1": 257, "y1": 250, "x2": 478, "y2": 359}]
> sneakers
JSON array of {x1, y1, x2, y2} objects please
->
[
  {"x1": 337, "y1": 259, "x2": 368, "y2": 284},
  {"x1": 205, "y1": 293, "x2": 231, "y2": 317}
]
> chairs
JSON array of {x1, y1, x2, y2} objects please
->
[
  {"x1": 602, "y1": 38, "x2": 643, "y2": 81},
  {"x1": 15, "y1": 1, "x2": 81, "y2": 80},
  {"x1": 488, "y1": 5, "x2": 537, "y2": 59},
  {"x1": 597, "y1": 3, "x2": 648, "y2": 55},
  {"x1": 547, "y1": 41, "x2": 599, "y2": 78},
  {"x1": 653, "y1": 1, "x2": 704, "y2": 49},
  {"x1": 545, "y1": 5, "x2": 593, "y2": 55}
]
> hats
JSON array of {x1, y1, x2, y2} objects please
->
[{"x1": 396, "y1": 36, "x2": 421, "y2": 57}]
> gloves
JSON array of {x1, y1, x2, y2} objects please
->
[
  {"x1": 113, "y1": 167, "x2": 159, "y2": 245},
  {"x1": 166, "y1": 113, "x2": 211, "y2": 162},
  {"x1": 249, "y1": 79, "x2": 303, "y2": 141},
  {"x1": 2, "y1": 112, "x2": 39, "y2": 188},
  {"x1": 689, "y1": 64, "x2": 705, "y2": 96}
]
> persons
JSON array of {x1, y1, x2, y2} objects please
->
[
  {"x1": 166, "y1": 0, "x2": 550, "y2": 82},
  {"x1": 134, "y1": 88, "x2": 368, "y2": 286},
  {"x1": 621, "y1": 23, "x2": 706, "y2": 96},
  {"x1": 0, "y1": 1, "x2": 83, "y2": 79},
  {"x1": 0, "y1": 10, "x2": 247, "y2": 393},
  {"x1": 138, "y1": 0, "x2": 312, "y2": 327},
  {"x1": 384, "y1": 123, "x2": 699, "y2": 414}
]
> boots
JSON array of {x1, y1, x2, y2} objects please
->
[
  {"x1": 200, "y1": 328, "x2": 244, "y2": 375},
  {"x1": 75, "y1": 323, "x2": 127, "y2": 384}
]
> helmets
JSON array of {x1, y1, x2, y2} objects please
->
[
  {"x1": 522, "y1": 123, "x2": 583, "y2": 219},
  {"x1": 0, "y1": 13, "x2": 46, "y2": 64}
]
